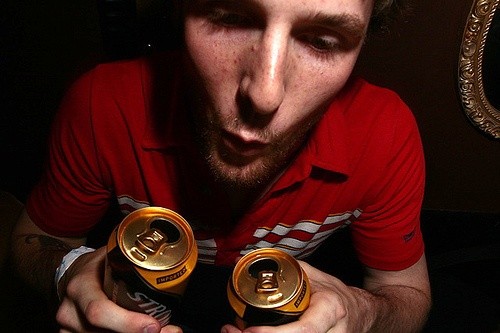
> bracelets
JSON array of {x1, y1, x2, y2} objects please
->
[{"x1": 53, "y1": 245, "x2": 98, "y2": 303}]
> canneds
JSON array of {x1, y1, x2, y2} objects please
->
[
  {"x1": 102, "y1": 206, "x2": 198, "y2": 327},
  {"x1": 225, "y1": 248, "x2": 312, "y2": 328}
]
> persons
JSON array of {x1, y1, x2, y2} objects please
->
[{"x1": 4, "y1": 1, "x2": 431, "y2": 333}]
